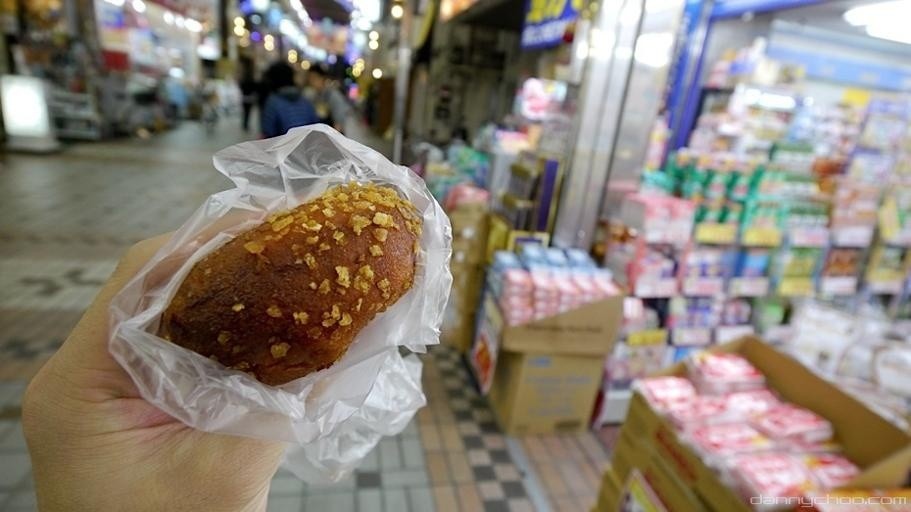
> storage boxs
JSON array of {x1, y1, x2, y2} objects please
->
[{"x1": 444, "y1": 202, "x2": 911, "y2": 512}]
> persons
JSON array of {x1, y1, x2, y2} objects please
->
[
  {"x1": 233, "y1": 50, "x2": 354, "y2": 141},
  {"x1": 20, "y1": 190, "x2": 345, "y2": 511}
]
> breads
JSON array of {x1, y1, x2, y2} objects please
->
[{"x1": 160, "y1": 180, "x2": 425, "y2": 387}]
